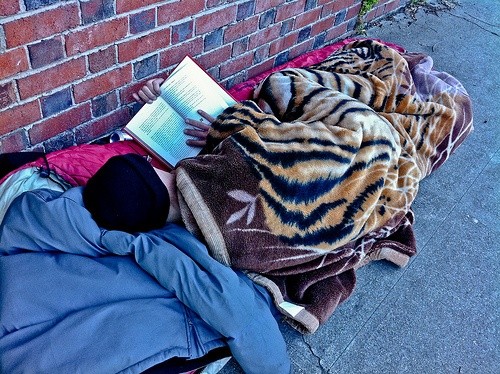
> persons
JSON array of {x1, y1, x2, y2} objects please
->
[{"x1": 81, "y1": 78, "x2": 217, "y2": 234}]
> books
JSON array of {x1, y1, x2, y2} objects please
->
[{"x1": 123, "y1": 54, "x2": 237, "y2": 170}]
